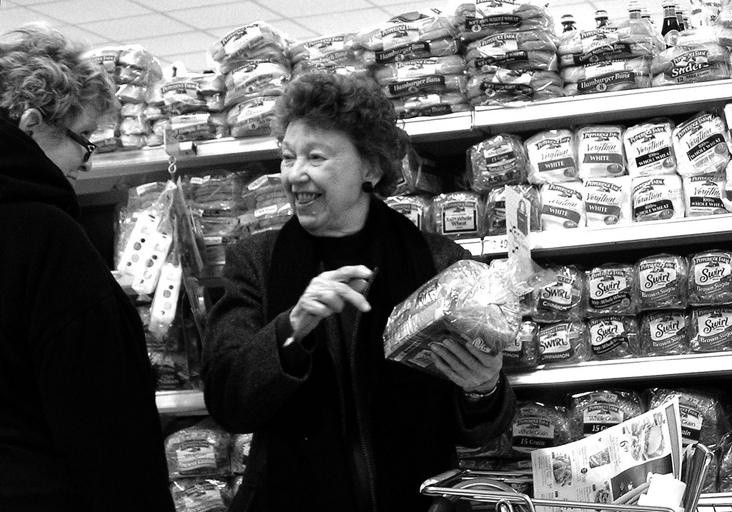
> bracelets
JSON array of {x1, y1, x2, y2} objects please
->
[{"x1": 462, "y1": 384, "x2": 498, "y2": 402}]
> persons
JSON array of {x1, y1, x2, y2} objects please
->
[
  {"x1": 0, "y1": 22, "x2": 184, "y2": 512},
  {"x1": 195, "y1": 67, "x2": 520, "y2": 510}
]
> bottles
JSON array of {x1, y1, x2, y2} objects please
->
[{"x1": 560, "y1": 0, "x2": 694, "y2": 50}]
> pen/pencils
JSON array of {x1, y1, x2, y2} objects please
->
[{"x1": 283, "y1": 279, "x2": 368, "y2": 348}]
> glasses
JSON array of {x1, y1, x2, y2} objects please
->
[{"x1": 41, "y1": 109, "x2": 98, "y2": 160}]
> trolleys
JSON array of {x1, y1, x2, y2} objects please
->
[{"x1": 418, "y1": 441, "x2": 731, "y2": 512}]
